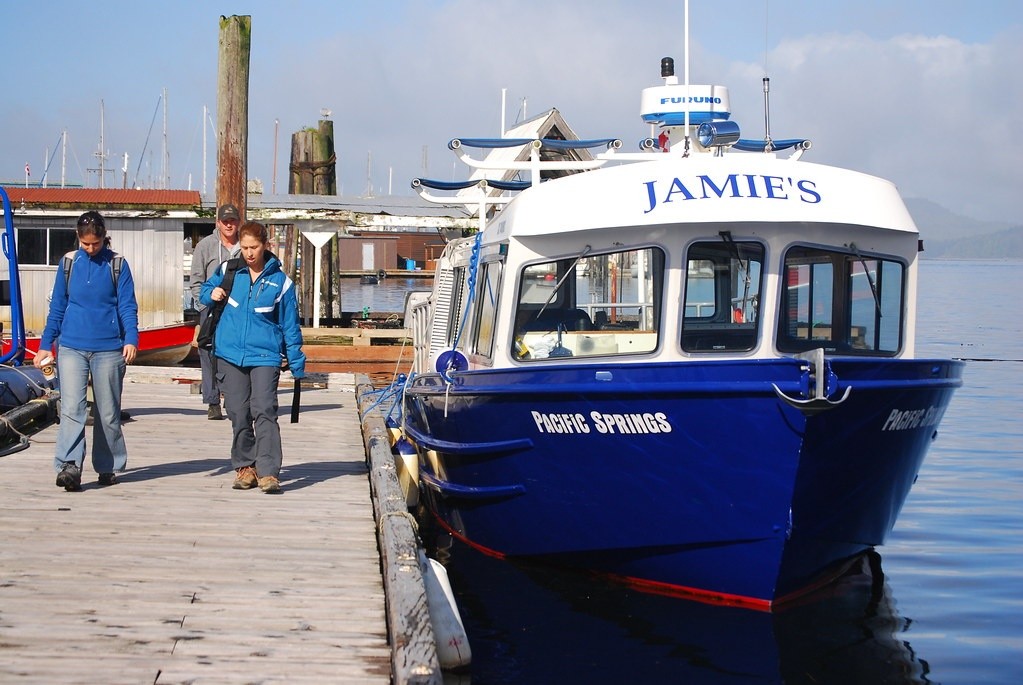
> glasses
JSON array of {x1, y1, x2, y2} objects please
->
[{"x1": 78, "y1": 216, "x2": 103, "y2": 226}]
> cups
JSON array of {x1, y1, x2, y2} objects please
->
[
  {"x1": 407, "y1": 260, "x2": 414, "y2": 270},
  {"x1": 40, "y1": 356, "x2": 56, "y2": 381}
]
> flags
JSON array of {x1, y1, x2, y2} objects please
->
[{"x1": 24, "y1": 163, "x2": 30, "y2": 176}]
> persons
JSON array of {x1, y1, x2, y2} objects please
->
[
  {"x1": 199, "y1": 220, "x2": 307, "y2": 492},
  {"x1": 189, "y1": 204, "x2": 245, "y2": 419},
  {"x1": 32, "y1": 211, "x2": 139, "y2": 491}
]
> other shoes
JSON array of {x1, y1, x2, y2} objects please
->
[
  {"x1": 98, "y1": 473, "x2": 117, "y2": 485},
  {"x1": 55, "y1": 467, "x2": 81, "y2": 492},
  {"x1": 208, "y1": 405, "x2": 222, "y2": 420}
]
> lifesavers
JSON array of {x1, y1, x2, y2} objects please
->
[
  {"x1": 420, "y1": 557, "x2": 473, "y2": 670},
  {"x1": 377, "y1": 269, "x2": 387, "y2": 279}
]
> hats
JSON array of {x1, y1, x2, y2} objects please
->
[{"x1": 218, "y1": 204, "x2": 240, "y2": 221}]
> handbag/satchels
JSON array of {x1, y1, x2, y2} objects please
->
[{"x1": 197, "y1": 259, "x2": 238, "y2": 350}]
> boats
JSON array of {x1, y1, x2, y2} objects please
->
[
  {"x1": 630, "y1": 250, "x2": 647, "y2": 277},
  {"x1": 576, "y1": 264, "x2": 590, "y2": 276},
  {"x1": 0, "y1": 319, "x2": 200, "y2": 368},
  {"x1": 536, "y1": 275, "x2": 557, "y2": 287},
  {"x1": 397, "y1": 57, "x2": 964, "y2": 613}
]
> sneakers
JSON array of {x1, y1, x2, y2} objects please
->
[
  {"x1": 258, "y1": 477, "x2": 280, "y2": 492},
  {"x1": 232, "y1": 466, "x2": 258, "y2": 489}
]
